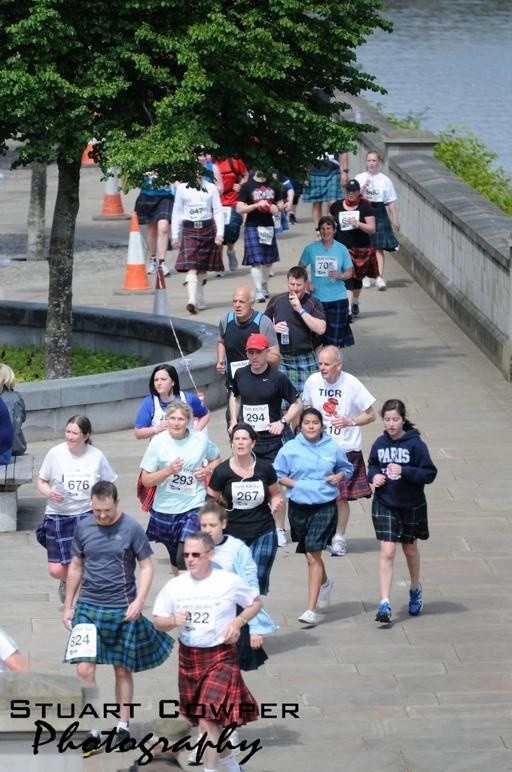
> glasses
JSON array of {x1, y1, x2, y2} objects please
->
[{"x1": 182, "y1": 552, "x2": 208, "y2": 558}]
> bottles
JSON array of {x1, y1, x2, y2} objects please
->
[
  {"x1": 280, "y1": 322, "x2": 291, "y2": 346},
  {"x1": 52, "y1": 479, "x2": 68, "y2": 508},
  {"x1": 328, "y1": 263, "x2": 335, "y2": 284}
]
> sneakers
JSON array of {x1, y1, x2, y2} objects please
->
[
  {"x1": 315, "y1": 227, "x2": 387, "y2": 324},
  {"x1": 80, "y1": 732, "x2": 103, "y2": 756},
  {"x1": 189, "y1": 729, "x2": 244, "y2": 771},
  {"x1": 59, "y1": 580, "x2": 67, "y2": 603},
  {"x1": 108, "y1": 729, "x2": 131, "y2": 752},
  {"x1": 331, "y1": 534, "x2": 347, "y2": 557},
  {"x1": 145, "y1": 214, "x2": 297, "y2": 315},
  {"x1": 407, "y1": 583, "x2": 422, "y2": 616},
  {"x1": 276, "y1": 528, "x2": 289, "y2": 547},
  {"x1": 375, "y1": 601, "x2": 393, "y2": 623},
  {"x1": 298, "y1": 610, "x2": 320, "y2": 624},
  {"x1": 318, "y1": 578, "x2": 333, "y2": 609}
]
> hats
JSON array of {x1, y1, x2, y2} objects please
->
[
  {"x1": 245, "y1": 334, "x2": 269, "y2": 351},
  {"x1": 345, "y1": 179, "x2": 360, "y2": 190}
]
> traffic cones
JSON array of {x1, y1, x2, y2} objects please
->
[
  {"x1": 195, "y1": 391, "x2": 210, "y2": 432},
  {"x1": 82, "y1": 109, "x2": 101, "y2": 168},
  {"x1": 111, "y1": 211, "x2": 157, "y2": 297},
  {"x1": 90, "y1": 164, "x2": 128, "y2": 222}
]
